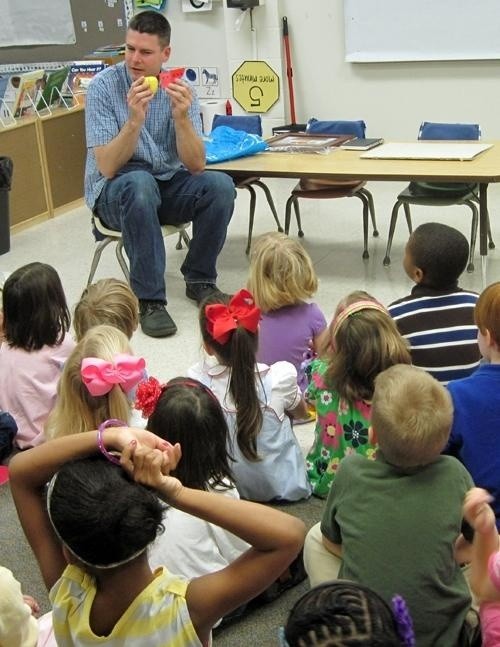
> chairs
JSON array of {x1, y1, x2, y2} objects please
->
[
  {"x1": 174, "y1": 113, "x2": 282, "y2": 255},
  {"x1": 284, "y1": 116, "x2": 380, "y2": 260},
  {"x1": 382, "y1": 121, "x2": 496, "y2": 273}
]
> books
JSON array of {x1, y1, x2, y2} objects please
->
[{"x1": 339, "y1": 137, "x2": 384, "y2": 152}]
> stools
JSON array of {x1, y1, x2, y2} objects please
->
[{"x1": 80, "y1": 206, "x2": 189, "y2": 293}]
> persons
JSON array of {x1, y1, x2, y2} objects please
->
[
  {"x1": 387, "y1": 222, "x2": 482, "y2": 386},
  {"x1": 244, "y1": 231, "x2": 328, "y2": 426},
  {"x1": 82, "y1": 9, "x2": 239, "y2": 339},
  {"x1": 2, "y1": 261, "x2": 306, "y2": 647},
  {"x1": 280, "y1": 285, "x2": 499, "y2": 647}
]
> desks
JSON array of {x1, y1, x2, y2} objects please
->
[{"x1": 204, "y1": 136, "x2": 500, "y2": 256}]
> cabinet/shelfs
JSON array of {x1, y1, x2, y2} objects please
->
[
  {"x1": 38, "y1": 104, "x2": 93, "y2": 211},
  {"x1": 1, "y1": 123, "x2": 49, "y2": 229}
]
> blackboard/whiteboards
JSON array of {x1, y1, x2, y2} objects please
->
[{"x1": 345, "y1": 0, "x2": 500, "y2": 62}]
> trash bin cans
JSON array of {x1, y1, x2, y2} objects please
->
[{"x1": 0, "y1": 156, "x2": 13, "y2": 255}]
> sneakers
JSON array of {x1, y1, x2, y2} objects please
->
[
  {"x1": 186, "y1": 283, "x2": 221, "y2": 306},
  {"x1": 139, "y1": 300, "x2": 177, "y2": 337}
]
